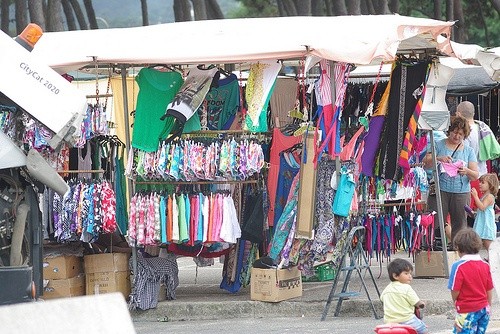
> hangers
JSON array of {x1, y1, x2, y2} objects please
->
[{"x1": 0, "y1": 48, "x2": 465, "y2": 222}]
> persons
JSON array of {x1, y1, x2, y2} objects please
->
[
  {"x1": 421, "y1": 118, "x2": 480, "y2": 251},
  {"x1": 447, "y1": 228, "x2": 494, "y2": 334},
  {"x1": 380, "y1": 258, "x2": 428, "y2": 334},
  {"x1": 455, "y1": 100, "x2": 488, "y2": 211},
  {"x1": 471, "y1": 173, "x2": 499, "y2": 262}
]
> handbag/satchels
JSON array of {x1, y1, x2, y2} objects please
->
[{"x1": 428, "y1": 175, "x2": 440, "y2": 196}]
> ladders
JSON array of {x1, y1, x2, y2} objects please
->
[{"x1": 320, "y1": 226, "x2": 384, "y2": 321}]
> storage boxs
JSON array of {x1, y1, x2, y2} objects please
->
[
  {"x1": 250, "y1": 263, "x2": 302, "y2": 303},
  {"x1": 300, "y1": 261, "x2": 335, "y2": 282},
  {"x1": 43, "y1": 231, "x2": 133, "y2": 303},
  {"x1": 414, "y1": 250, "x2": 462, "y2": 278}
]
